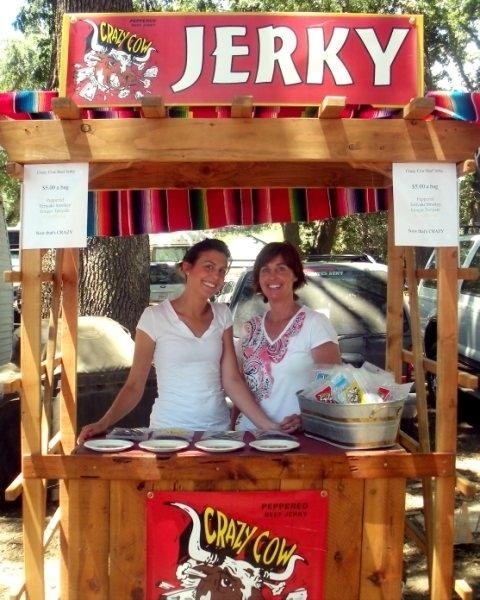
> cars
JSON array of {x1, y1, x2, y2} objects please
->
[{"x1": 149, "y1": 242, "x2": 412, "y2": 380}]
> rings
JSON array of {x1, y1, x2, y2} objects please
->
[{"x1": 293, "y1": 423, "x2": 298, "y2": 429}]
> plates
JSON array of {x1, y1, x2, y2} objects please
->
[{"x1": 85, "y1": 439, "x2": 300, "y2": 453}]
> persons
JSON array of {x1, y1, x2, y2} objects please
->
[
  {"x1": 75, "y1": 238, "x2": 286, "y2": 450},
  {"x1": 227, "y1": 240, "x2": 344, "y2": 436}
]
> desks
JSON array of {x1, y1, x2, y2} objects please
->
[{"x1": 59, "y1": 431, "x2": 407, "y2": 600}]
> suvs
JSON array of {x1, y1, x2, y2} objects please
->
[{"x1": 418, "y1": 234, "x2": 480, "y2": 364}]
